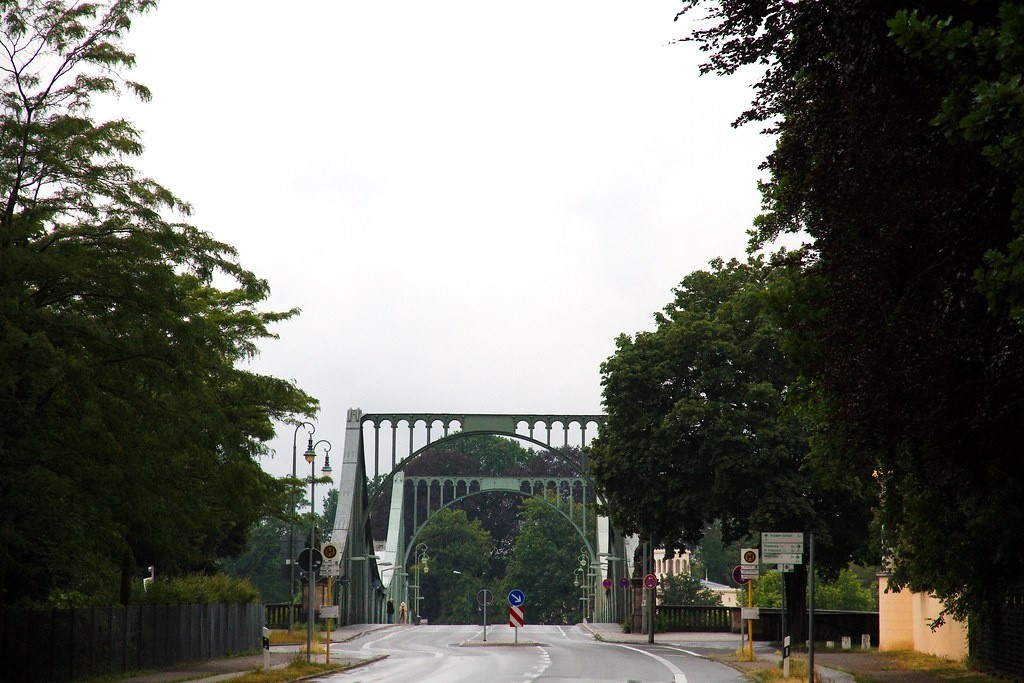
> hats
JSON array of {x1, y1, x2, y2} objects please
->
[
  {"x1": 389, "y1": 598, "x2": 394, "y2": 600},
  {"x1": 401, "y1": 602, "x2": 405, "y2": 606}
]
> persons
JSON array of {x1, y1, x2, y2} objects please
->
[
  {"x1": 387, "y1": 597, "x2": 394, "y2": 624},
  {"x1": 398, "y1": 602, "x2": 408, "y2": 625}
]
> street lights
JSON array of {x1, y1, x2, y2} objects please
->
[
  {"x1": 414, "y1": 543, "x2": 430, "y2": 616},
  {"x1": 303, "y1": 440, "x2": 332, "y2": 664},
  {"x1": 288, "y1": 421, "x2": 317, "y2": 632},
  {"x1": 344, "y1": 555, "x2": 409, "y2": 626},
  {"x1": 573, "y1": 545, "x2": 627, "y2": 624}
]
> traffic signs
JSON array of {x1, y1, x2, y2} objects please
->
[
  {"x1": 761, "y1": 532, "x2": 803, "y2": 544},
  {"x1": 762, "y1": 542, "x2": 804, "y2": 554},
  {"x1": 761, "y1": 554, "x2": 802, "y2": 564}
]
